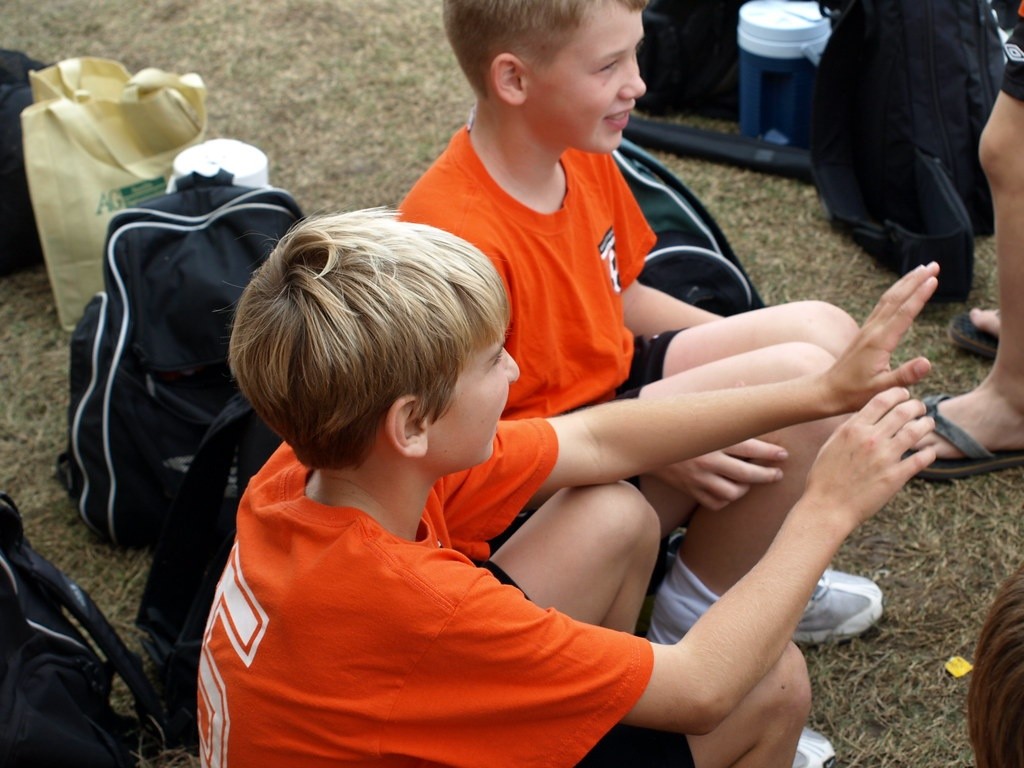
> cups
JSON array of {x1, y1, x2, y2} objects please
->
[
  {"x1": 736, "y1": 0, "x2": 833, "y2": 148},
  {"x1": 165, "y1": 138, "x2": 273, "y2": 196}
]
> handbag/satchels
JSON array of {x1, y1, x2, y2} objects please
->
[
  {"x1": 0, "y1": 48, "x2": 55, "y2": 277},
  {"x1": 611, "y1": 135, "x2": 767, "y2": 400},
  {"x1": 19, "y1": 57, "x2": 211, "y2": 335}
]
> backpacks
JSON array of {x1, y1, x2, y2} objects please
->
[
  {"x1": 0, "y1": 497, "x2": 170, "y2": 768},
  {"x1": 810, "y1": 0, "x2": 1005, "y2": 304},
  {"x1": 53, "y1": 168, "x2": 314, "y2": 545}
]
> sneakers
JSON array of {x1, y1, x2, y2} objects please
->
[
  {"x1": 791, "y1": 727, "x2": 835, "y2": 768},
  {"x1": 646, "y1": 551, "x2": 885, "y2": 647}
]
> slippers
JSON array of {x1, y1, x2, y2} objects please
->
[
  {"x1": 948, "y1": 311, "x2": 999, "y2": 357},
  {"x1": 900, "y1": 395, "x2": 1024, "y2": 481}
]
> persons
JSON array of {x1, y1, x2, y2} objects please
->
[
  {"x1": 967, "y1": 560, "x2": 1024, "y2": 768},
  {"x1": 893, "y1": 0, "x2": 1024, "y2": 479},
  {"x1": 197, "y1": 210, "x2": 940, "y2": 768},
  {"x1": 396, "y1": 0, "x2": 884, "y2": 645}
]
